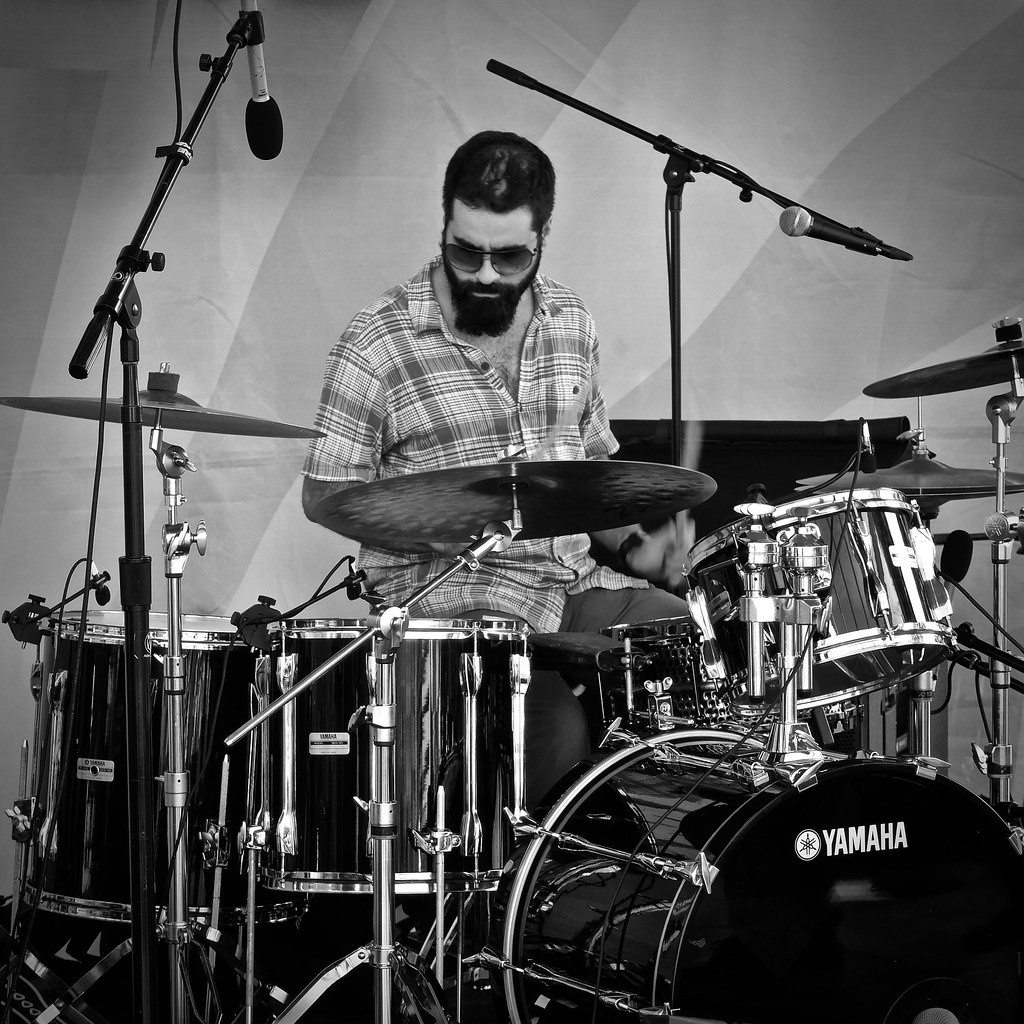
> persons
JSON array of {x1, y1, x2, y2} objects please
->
[{"x1": 301, "y1": 130, "x2": 694, "y2": 812}]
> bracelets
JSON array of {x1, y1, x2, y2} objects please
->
[{"x1": 617, "y1": 530, "x2": 651, "y2": 571}]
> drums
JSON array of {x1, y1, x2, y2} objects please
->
[
  {"x1": 258, "y1": 611, "x2": 530, "y2": 901},
  {"x1": 492, "y1": 732, "x2": 1023, "y2": 1024},
  {"x1": 683, "y1": 484, "x2": 959, "y2": 716},
  {"x1": 15, "y1": 610, "x2": 264, "y2": 934}
]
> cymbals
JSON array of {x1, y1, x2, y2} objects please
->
[
  {"x1": 307, "y1": 457, "x2": 726, "y2": 553},
  {"x1": 792, "y1": 452, "x2": 1024, "y2": 509},
  {"x1": 0, "y1": 367, "x2": 331, "y2": 449},
  {"x1": 864, "y1": 338, "x2": 1024, "y2": 398}
]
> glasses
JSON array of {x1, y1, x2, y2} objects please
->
[{"x1": 441, "y1": 243, "x2": 540, "y2": 276}]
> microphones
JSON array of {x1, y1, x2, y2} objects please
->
[
  {"x1": 913, "y1": 1008, "x2": 960, "y2": 1024},
  {"x1": 346, "y1": 557, "x2": 363, "y2": 600},
  {"x1": 90, "y1": 560, "x2": 110, "y2": 606},
  {"x1": 860, "y1": 418, "x2": 876, "y2": 474},
  {"x1": 241, "y1": 0, "x2": 284, "y2": 160},
  {"x1": 940, "y1": 530, "x2": 973, "y2": 603},
  {"x1": 779, "y1": 206, "x2": 914, "y2": 261}
]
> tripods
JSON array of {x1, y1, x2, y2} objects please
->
[{"x1": 0, "y1": 428, "x2": 532, "y2": 1024}]
